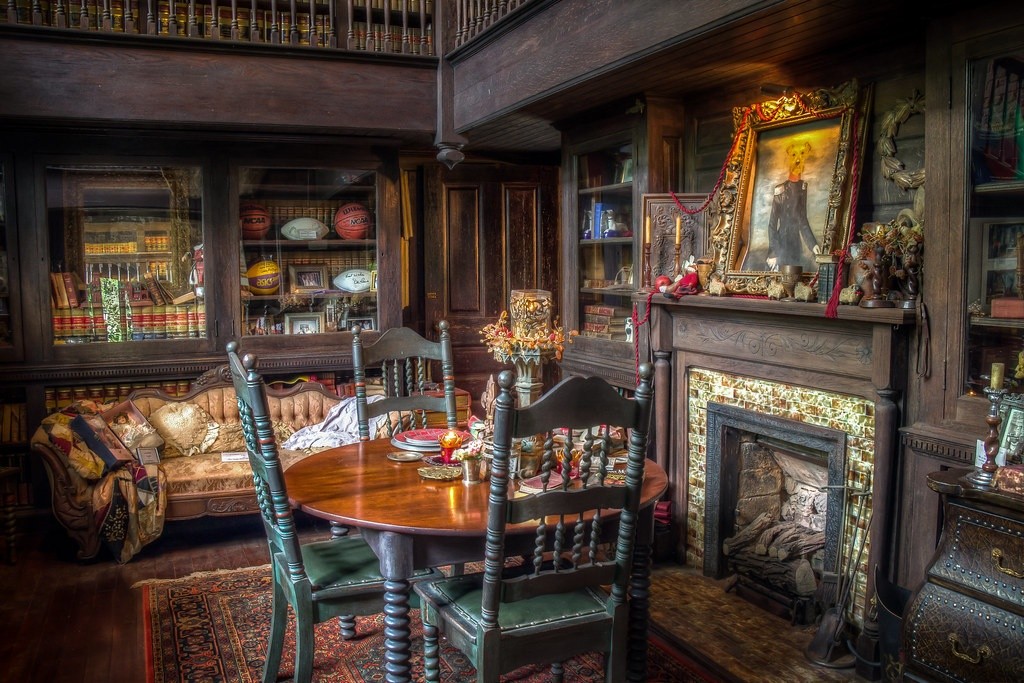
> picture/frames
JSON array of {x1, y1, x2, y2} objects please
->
[
  {"x1": 370, "y1": 269, "x2": 378, "y2": 291},
  {"x1": 346, "y1": 317, "x2": 375, "y2": 331},
  {"x1": 287, "y1": 263, "x2": 329, "y2": 294},
  {"x1": 997, "y1": 406, "x2": 1024, "y2": 456},
  {"x1": 704, "y1": 75, "x2": 873, "y2": 299},
  {"x1": 259, "y1": 314, "x2": 275, "y2": 335},
  {"x1": 285, "y1": 312, "x2": 325, "y2": 335},
  {"x1": 245, "y1": 316, "x2": 260, "y2": 336}
]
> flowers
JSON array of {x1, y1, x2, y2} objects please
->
[{"x1": 449, "y1": 439, "x2": 483, "y2": 462}]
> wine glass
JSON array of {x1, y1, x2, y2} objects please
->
[{"x1": 779, "y1": 265, "x2": 803, "y2": 302}]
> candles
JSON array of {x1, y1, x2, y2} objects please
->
[
  {"x1": 675, "y1": 214, "x2": 682, "y2": 244},
  {"x1": 645, "y1": 214, "x2": 651, "y2": 243},
  {"x1": 990, "y1": 362, "x2": 1004, "y2": 390}
]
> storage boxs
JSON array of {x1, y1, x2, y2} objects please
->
[
  {"x1": 584, "y1": 248, "x2": 605, "y2": 280},
  {"x1": 603, "y1": 244, "x2": 622, "y2": 280},
  {"x1": 990, "y1": 463, "x2": 1024, "y2": 497},
  {"x1": 135, "y1": 446, "x2": 162, "y2": 467},
  {"x1": 982, "y1": 223, "x2": 1019, "y2": 315},
  {"x1": 70, "y1": 399, "x2": 166, "y2": 473},
  {"x1": 989, "y1": 296, "x2": 1024, "y2": 319},
  {"x1": 583, "y1": 279, "x2": 622, "y2": 288}
]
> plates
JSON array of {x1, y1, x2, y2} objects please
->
[
  {"x1": 386, "y1": 452, "x2": 424, "y2": 462},
  {"x1": 390, "y1": 429, "x2": 477, "y2": 452},
  {"x1": 417, "y1": 467, "x2": 461, "y2": 479}
]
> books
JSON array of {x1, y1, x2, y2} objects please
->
[
  {"x1": 974, "y1": 56, "x2": 1024, "y2": 179},
  {"x1": 238, "y1": 196, "x2": 380, "y2": 335},
  {"x1": 990, "y1": 296, "x2": 1024, "y2": 319},
  {"x1": 579, "y1": 155, "x2": 634, "y2": 342},
  {"x1": 49, "y1": 272, "x2": 207, "y2": 343}
]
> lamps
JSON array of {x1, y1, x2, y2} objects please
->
[{"x1": 478, "y1": 289, "x2": 579, "y2": 407}]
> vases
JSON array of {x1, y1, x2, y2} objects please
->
[{"x1": 460, "y1": 459, "x2": 482, "y2": 486}]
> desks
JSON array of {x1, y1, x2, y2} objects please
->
[{"x1": 282, "y1": 431, "x2": 668, "y2": 683}]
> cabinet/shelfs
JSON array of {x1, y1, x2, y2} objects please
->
[
  {"x1": 412, "y1": 383, "x2": 472, "y2": 430},
  {"x1": 567, "y1": 119, "x2": 640, "y2": 364},
  {"x1": 60, "y1": 163, "x2": 192, "y2": 298},
  {"x1": 899, "y1": 467, "x2": 1024, "y2": 683},
  {"x1": 18, "y1": 150, "x2": 403, "y2": 369},
  {"x1": 942, "y1": 24, "x2": 1024, "y2": 438}
]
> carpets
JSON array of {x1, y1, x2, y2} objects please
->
[{"x1": 129, "y1": 562, "x2": 748, "y2": 683}]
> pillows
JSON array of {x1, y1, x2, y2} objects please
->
[{"x1": 148, "y1": 400, "x2": 220, "y2": 458}]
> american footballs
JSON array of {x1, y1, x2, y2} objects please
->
[
  {"x1": 280, "y1": 219, "x2": 330, "y2": 240},
  {"x1": 333, "y1": 269, "x2": 372, "y2": 293}
]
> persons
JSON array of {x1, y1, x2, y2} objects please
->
[{"x1": 112, "y1": 411, "x2": 154, "y2": 447}]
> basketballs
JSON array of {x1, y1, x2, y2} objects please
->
[
  {"x1": 335, "y1": 202, "x2": 374, "y2": 239},
  {"x1": 247, "y1": 258, "x2": 281, "y2": 295},
  {"x1": 240, "y1": 203, "x2": 271, "y2": 239}
]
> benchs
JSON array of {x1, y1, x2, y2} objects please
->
[{"x1": 23, "y1": 363, "x2": 414, "y2": 560}]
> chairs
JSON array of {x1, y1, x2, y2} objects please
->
[
  {"x1": 224, "y1": 341, "x2": 448, "y2": 683},
  {"x1": 410, "y1": 361, "x2": 657, "y2": 683},
  {"x1": 350, "y1": 318, "x2": 466, "y2": 578}
]
